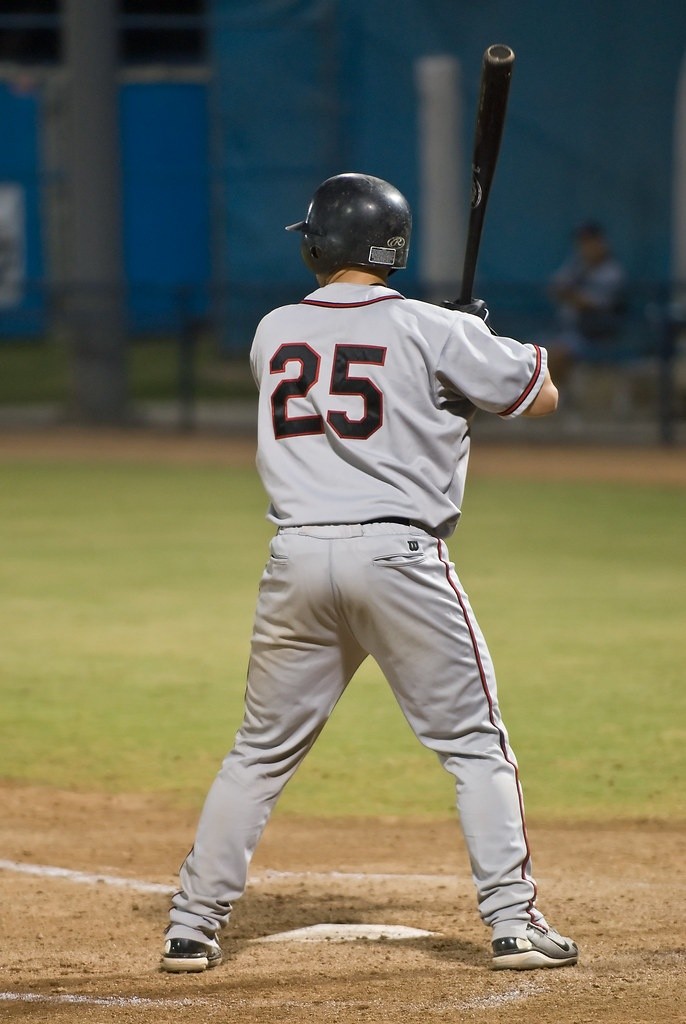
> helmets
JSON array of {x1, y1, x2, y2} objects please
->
[{"x1": 283, "y1": 172, "x2": 410, "y2": 275}]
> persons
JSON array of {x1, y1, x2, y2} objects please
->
[
  {"x1": 537, "y1": 221, "x2": 640, "y2": 366},
  {"x1": 159, "y1": 173, "x2": 580, "y2": 972}
]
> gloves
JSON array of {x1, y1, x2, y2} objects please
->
[{"x1": 440, "y1": 298, "x2": 487, "y2": 320}]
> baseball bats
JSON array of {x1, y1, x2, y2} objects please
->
[{"x1": 460, "y1": 44, "x2": 517, "y2": 306}]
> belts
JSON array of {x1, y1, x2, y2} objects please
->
[{"x1": 361, "y1": 517, "x2": 409, "y2": 526}]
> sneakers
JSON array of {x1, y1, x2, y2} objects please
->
[
  {"x1": 162, "y1": 939, "x2": 222, "y2": 973},
  {"x1": 489, "y1": 923, "x2": 579, "y2": 968}
]
